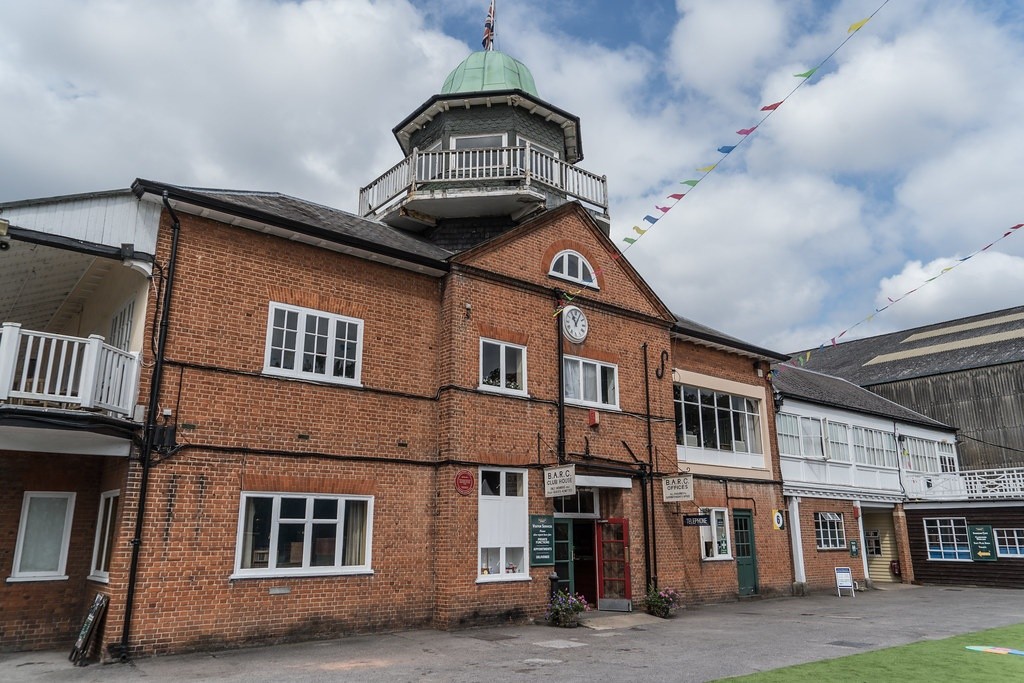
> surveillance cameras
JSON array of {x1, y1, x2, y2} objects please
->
[{"x1": 466, "y1": 304, "x2": 471, "y2": 316}]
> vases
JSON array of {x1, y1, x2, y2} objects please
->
[
  {"x1": 648, "y1": 606, "x2": 669, "y2": 617},
  {"x1": 555, "y1": 617, "x2": 578, "y2": 626}
]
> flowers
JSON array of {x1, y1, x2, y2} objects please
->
[
  {"x1": 642, "y1": 583, "x2": 681, "y2": 617},
  {"x1": 544, "y1": 587, "x2": 590, "y2": 619}
]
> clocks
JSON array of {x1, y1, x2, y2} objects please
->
[{"x1": 563, "y1": 304, "x2": 588, "y2": 343}]
